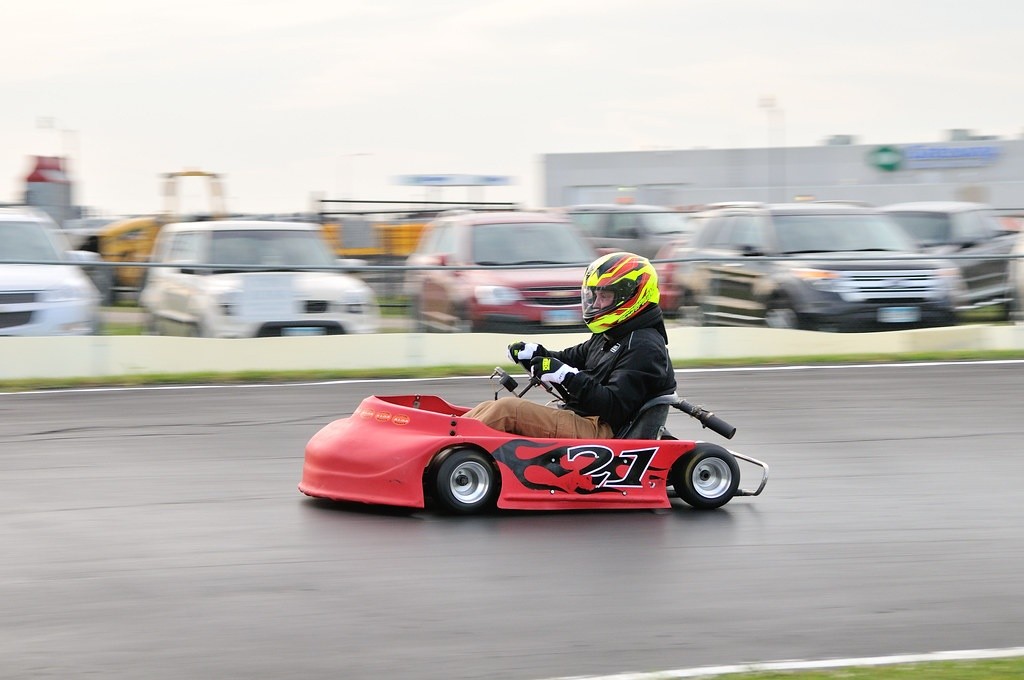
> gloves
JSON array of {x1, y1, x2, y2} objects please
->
[
  {"x1": 530, "y1": 357, "x2": 579, "y2": 384},
  {"x1": 508, "y1": 341, "x2": 552, "y2": 364}
]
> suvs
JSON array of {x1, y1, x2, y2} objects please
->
[
  {"x1": 878, "y1": 204, "x2": 1021, "y2": 320},
  {"x1": 406, "y1": 201, "x2": 601, "y2": 331},
  {"x1": 671, "y1": 202, "x2": 965, "y2": 334}
]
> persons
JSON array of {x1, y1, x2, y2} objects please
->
[{"x1": 460, "y1": 252, "x2": 676, "y2": 439}]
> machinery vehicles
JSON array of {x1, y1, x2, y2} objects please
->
[{"x1": 85, "y1": 173, "x2": 266, "y2": 307}]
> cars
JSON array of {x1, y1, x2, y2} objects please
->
[
  {"x1": 568, "y1": 201, "x2": 696, "y2": 266},
  {"x1": 0, "y1": 204, "x2": 102, "y2": 337},
  {"x1": 137, "y1": 218, "x2": 385, "y2": 335}
]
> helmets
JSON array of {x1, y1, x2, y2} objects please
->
[{"x1": 581, "y1": 252, "x2": 660, "y2": 334}]
